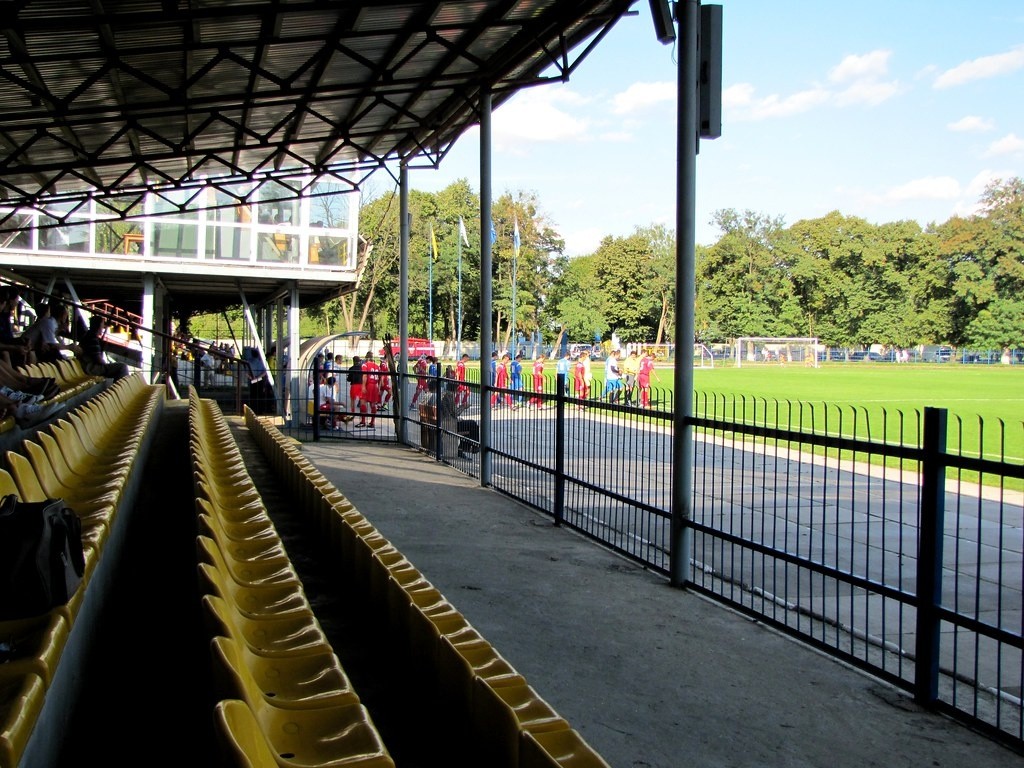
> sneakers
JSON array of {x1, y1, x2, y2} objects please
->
[
  {"x1": 17, "y1": 402, "x2": 66, "y2": 427},
  {"x1": 8, "y1": 391, "x2": 44, "y2": 406}
]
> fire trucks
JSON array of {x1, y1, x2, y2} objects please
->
[{"x1": 379, "y1": 336, "x2": 435, "y2": 362}]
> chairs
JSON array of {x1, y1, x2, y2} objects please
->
[{"x1": 0, "y1": 324, "x2": 609, "y2": 768}]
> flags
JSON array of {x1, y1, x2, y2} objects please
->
[
  {"x1": 514, "y1": 221, "x2": 520, "y2": 258},
  {"x1": 461, "y1": 221, "x2": 469, "y2": 247},
  {"x1": 491, "y1": 220, "x2": 496, "y2": 244},
  {"x1": 432, "y1": 228, "x2": 438, "y2": 259}
]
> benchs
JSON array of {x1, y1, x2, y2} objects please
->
[{"x1": 418, "y1": 404, "x2": 471, "y2": 453}]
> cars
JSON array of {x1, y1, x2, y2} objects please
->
[
  {"x1": 570, "y1": 344, "x2": 601, "y2": 361},
  {"x1": 818, "y1": 344, "x2": 980, "y2": 363}
]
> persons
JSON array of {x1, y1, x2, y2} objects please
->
[
  {"x1": 894, "y1": 348, "x2": 909, "y2": 363},
  {"x1": 807, "y1": 353, "x2": 814, "y2": 367},
  {"x1": 409, "y1": 355, "x2": 479, "y2": 460},
  {"x1": 309, "y1": 347, "x2": 400, "y2": 433},
  {"x1": 527, "y1": 354, "x2": 548, "y2": 410},
  {"x1": 244, "y1": 345, "x2": 276, "y2": 413},
  {"x1": 780, "y1": 353, "x2": 786, "y2": 368},
  {"x1": 492, "y1": 352, "x2": 524, "y2": 412},
  {"x1": 273, "y1": 214, "x2": 328, "y2": 254},
  {"x1": 0, "y1": 286, "x2": 129, "y2": 427},
  {"x1": 596, "y1": 349, "x2": 660, "y2": 410},
  {"x1": 556, "y1": 350, "x2": 591, "y2": 412},
  {"x1": 173, "y1": 325, "x2": 235, "y2": 386},
  {"x1": 762, "y1": 348, "x2": 772, "y2": 363},
  {"x1": 1002, "y1": 347, "x2": 1010, "y2": 364},
  {"x1": 455, "y1": 354, "x2": 471, "y2": 409}
]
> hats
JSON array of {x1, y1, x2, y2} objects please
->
[{"x1": 353, "y1": 356, "x2": 361, "y2": 362}]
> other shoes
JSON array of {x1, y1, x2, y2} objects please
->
[
  {"x1": 343, "y1": 416, "x2": 355, "y2": 425},
  {"x1": 333, "y1": 426, "x2": 342, "y2": 431},
  {"x1": 367, "y1": 422, "x2": 375, "y2": 428},
  {"x1": 377, "y1": 407, "x2": 386, "y2": 411},
  {"x1": 34, "y1": 379, "x2": 50, "y2": 395},
  {"x1": 410, "y1": 404, "x2": 417, "y2": 410},
  {"x1": 355, "y1": 422, "x2": 366, "y2": 427},
  {"x1": 383, "y1": 404, "x2": 389, "y2": 411}
]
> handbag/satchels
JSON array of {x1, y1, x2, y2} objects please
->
[{"x1": 0, "y1": 494, "x2": 85, "y2": 621}]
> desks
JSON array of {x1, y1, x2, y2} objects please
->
[{"x1": 122, "y1": 234, "x2": 145, "y2": 255}]
